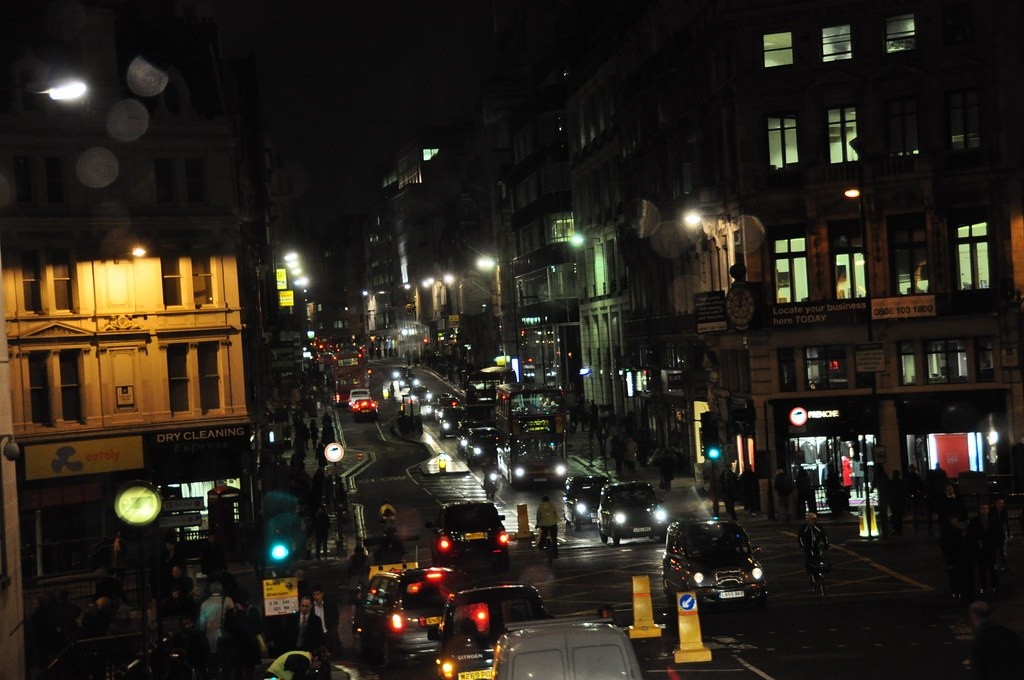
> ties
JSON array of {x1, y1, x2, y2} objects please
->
[{"x1": 301, "y1": 616, "x2": 307, "y2": 629}]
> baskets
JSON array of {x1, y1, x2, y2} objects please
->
[{"x1": 810, "y1": 560, "x2": 832, "y2": 574}]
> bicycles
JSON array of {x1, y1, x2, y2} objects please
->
[{"x1": 535, "y1": 524, "x2": 558, "y2": 565}]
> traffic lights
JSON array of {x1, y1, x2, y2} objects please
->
[
  {"x1": 706, "y1": 445, "x2": 722, "y2": 462},
  {"x1": 267, "y1": 539, "x2": 293, "y2": 563}
]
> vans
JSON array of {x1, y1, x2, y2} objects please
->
[
  {"x1": 663, "y1": 519, "x2": 769, "y2": 610},
  {"x1": 427, "y1": 585, "x2": 643, "y2": 680}
]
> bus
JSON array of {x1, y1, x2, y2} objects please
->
[
  {"x1": 495, "y1": 383, "x2": 568, "y2": 488},
  {"x1": 331, "y1": 353, "x2": 369, "y2": 392}
]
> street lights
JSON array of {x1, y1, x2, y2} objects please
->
[{"x1": 255, "y1": 250, "x2": 308, "y2": 345}]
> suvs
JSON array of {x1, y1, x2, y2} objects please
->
[
  {"x1": 425, "y1": 500, "x2": 510, "y2": 570},
  {"x1": 348, "y1": 388, "x2": 378, "y2": 422},
  {"x1": 351, "y1": 566, "x2": 489, "y2": 677},
  {"x1": 432, "y1": 393, "x2": 496, "y2": 466},
  {"x1": 563, "y1": 476, "x2": 669, "y2": 545}
]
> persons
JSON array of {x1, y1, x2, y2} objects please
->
[
  {"x1": 774, "y1": 466, "x2": 842, "y2": 520},
  {"x1": 938, "y1": 483, "x2": 1013, "y2": 573},
  {"x1": 348, "y1": 523, "x2": 405, "y2": 590},
  {"x1": 720, "y1": 463, "x2": 759, "y2": 520},
  {"x1": 570, "y1": 399, "x2": 649, "y2": 479},
  {"x1": 873, "y1": 464, "x2": 921, "y2": 539},
  {"x1": 32, "y1": 535, "x2": 343, "y2": 680},
  {"x1": 536, "y1": 496, "x2": 562, "y2": 559},
  {"x1": 659, "y1": 450, "x2": 674, "y2": 493},
  {"x1": 798, "y1": 512, "x2": 829, "y2": 578},
  {"x1": 970, "y1": 602, "x2": 1024, "y2": 680},
  {"x1": 292, "y1": 413, "x2": 348, "y2": 560}
]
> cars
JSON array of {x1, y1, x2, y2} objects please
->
[{"x1": 391, "y1": 367, "x2": 438, "y2": 420}]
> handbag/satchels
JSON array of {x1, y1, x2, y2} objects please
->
[{"x1": 220, "y1": 596, "x2": 240, "y2": 638}]
